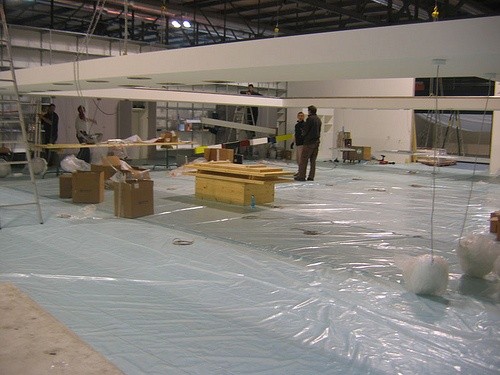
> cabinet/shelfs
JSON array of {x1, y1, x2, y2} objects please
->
[
  {"x1": 351, "y1": 146, "x2": 371, "y2": 159},
  {"x1": 303, "y1": 108, "x2": 334, "y2": 161}
]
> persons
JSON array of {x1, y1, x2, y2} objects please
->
[
  {"x1": 240, "y1": 84, "x2": 263, "y2": 138},
  {"x1": 294, "y1": 112, "x2": 305, "y2": 176},
  {"x1": 75, "y1": 105, "x2": 96, "y2": 162},
  {"x1": 39, "y1": 104, "x2": 59, "y2": 166},
  {"x1": 294, "y1": 106, "x2": 321, "y2": 181}
]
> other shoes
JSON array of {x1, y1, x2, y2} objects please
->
[
  {"x1": 307, "y1": 178, "x2": 313, "y2": 181},
  {"x1": 295, "y1": 178, "x2": 305, "y2": 181}
]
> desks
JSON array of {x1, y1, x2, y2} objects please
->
[
  {"x1": 329, "y1": 148, "x2": 355, "y2": 161},
  {"x1": 33, "y1": 142, "x2": 193, "y2": 179}
]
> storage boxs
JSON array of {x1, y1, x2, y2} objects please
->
[
  {"x1": 59, "y1": 174, "x2": 72, "y2": 199},
  {"x1": 113, "y1": 180, "x2": 154, "y2": 218},
  {"x1": 72, "y1": 170, "x2": 104, "y2": 203},
  {"x1": 120, "y1": 167, "x2": 151, "y2": 179},
  {"x1": 90, "y1": 156, "x2": 120, "y2": 181}
]
